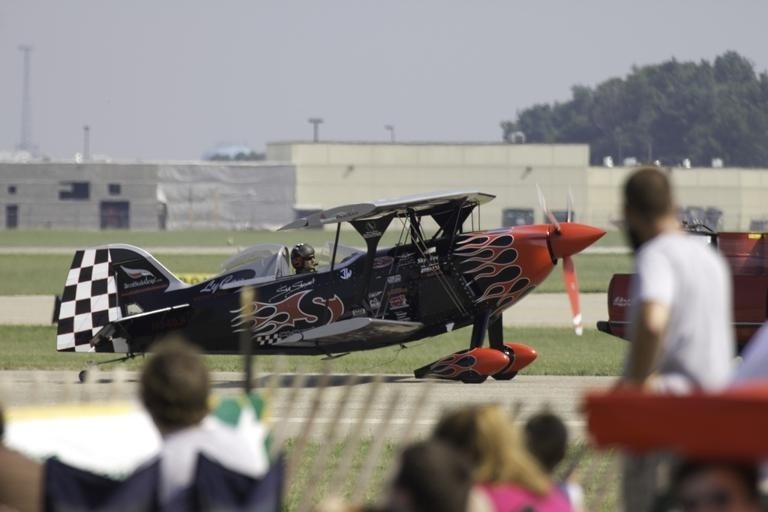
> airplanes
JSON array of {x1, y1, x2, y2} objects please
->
[{"x1": 51, "y1": 191, "x2": 608, "y2": 384}]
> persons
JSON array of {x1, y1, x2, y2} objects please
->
[
  {"x1": 389, "y1": 398, "x2": 586, "y2": 512},
  {"x1": 618, "y1": 168, "x2": 768, "y2": 511},
  {"x1": 290, "y1": 243, "x2": 318, "y2": 273},
  {"x1": 0, "y1": 344, "x2": 285, "y2": 510}
]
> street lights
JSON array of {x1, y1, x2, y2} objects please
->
[{"x1": 308, "y1": 117, "x2": 325, "y2": 142}]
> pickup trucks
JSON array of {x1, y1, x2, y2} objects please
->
[{"x1": 596, "y1": 273, "x2": 768, "y2": 357}]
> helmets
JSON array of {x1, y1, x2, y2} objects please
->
[{"x1": 291, "y1": 243, "x2": 315, "y2": 268}]
